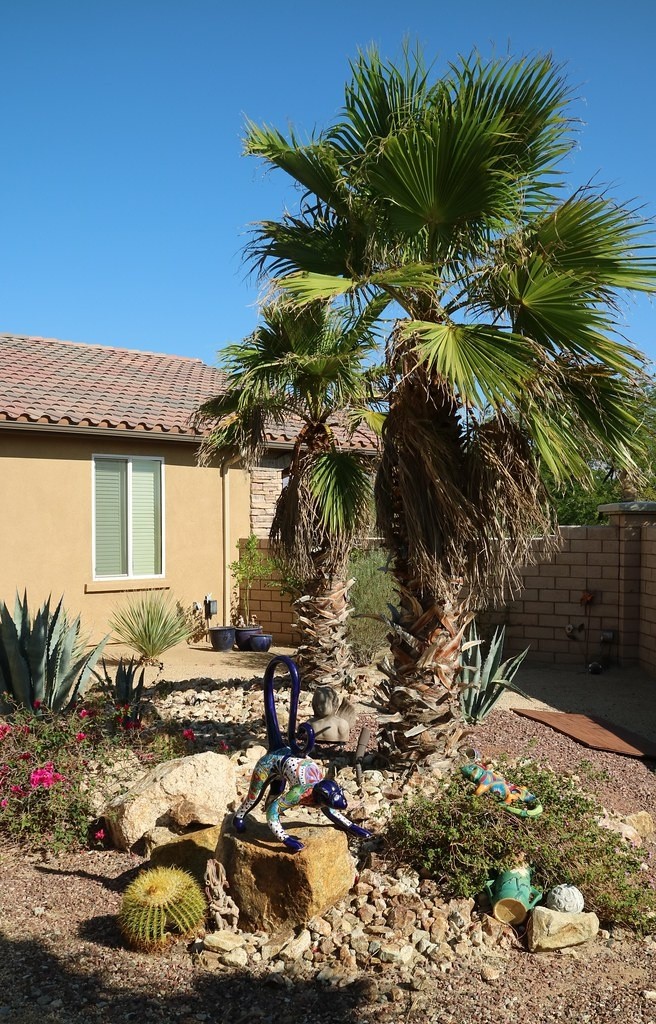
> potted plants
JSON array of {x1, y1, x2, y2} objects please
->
[{"x1": 230, "y1": 535, "x2": 274, "y2": 647}]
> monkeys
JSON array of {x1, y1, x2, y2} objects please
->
[{"x1": 232, "y1": 655, "x2": 376, "y2": 851}]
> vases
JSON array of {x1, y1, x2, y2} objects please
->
[
  {"x1": 209, "y1": 626, "x2": 234, "y2": 650},
  {"x1": 250, "y1": 633, "x2": 272, "y2": 652}
]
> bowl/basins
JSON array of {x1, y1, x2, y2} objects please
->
[
  {"x1": 208, "y1": 626, "x2": 236, "y2": 651},
  {"x1": 250, "y1": 634, "x2": 273, "y2": 652}
]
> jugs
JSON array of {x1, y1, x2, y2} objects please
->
[{"x1": 486, "y1": 864, "x2": 542, "y2": 925}]
